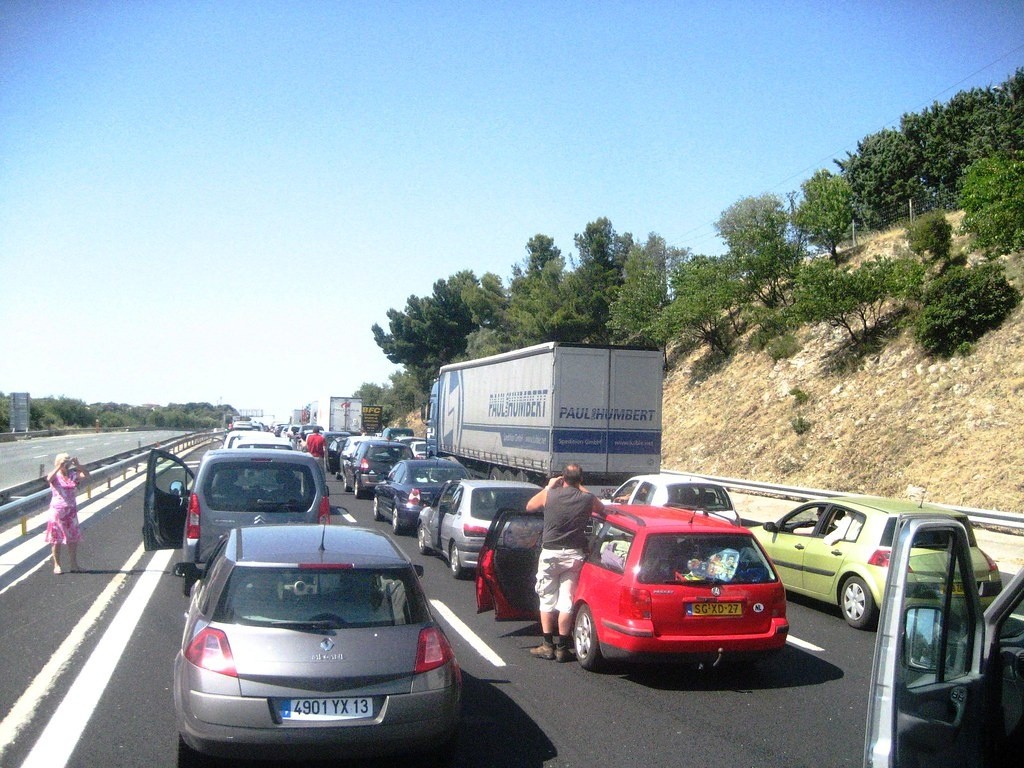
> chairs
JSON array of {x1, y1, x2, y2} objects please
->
[
  {"x1": 214, "y1": 472, "x2": 243, "y2": 494},
  {"x1": 679, "y1": 488, "x2": 717, "y2": 505},
  {"x1": 281, "y1": 575, "x2": 318, "y2": 605},
  {"x1": 342, "y1": 575, "x2": 382, "y2": 603},
  {"x1": 274, "y1": 470, "x2": 302, "y2": 498}
]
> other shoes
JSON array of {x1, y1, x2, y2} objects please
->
[
  {"x1": 54, "y1": 564, "x2": 62, "y2": 574},
  {"x1": 71, "y1": 566, "x2": 87, "y2": 572},
  {"x1": 555, "y1": 644, "x2": 576, "y2": 663},
  {"x1": 530, "y1": 641, "x2": 555, "y2": 659}
]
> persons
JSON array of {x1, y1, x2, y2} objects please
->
[
  {"x1": 524, "y1": 465, "x2": 604, "y2": 662},
  {"x1": 227, "y1": 420, "x2": 329, "y2": 471},
  {"x1": 711, "y1": 556, "x2": 735, "y2": 576},
  {"x1": 613, "y1": 479, "x2": 843, "y2": 538},
  {"x1": 46, "y1": 454, "x2": 86, "y2": 574},
  {"x1": 360, "y1": 422, "x2": 392, "y2": 440}
]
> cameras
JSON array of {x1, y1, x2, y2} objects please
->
[{"x1": 65, "y1": 459, "x2": 73, "y2": 465}]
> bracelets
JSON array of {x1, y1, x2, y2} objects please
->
[{"x1": 544, "y1": 485, "x2": 550, "y2": 491}]
